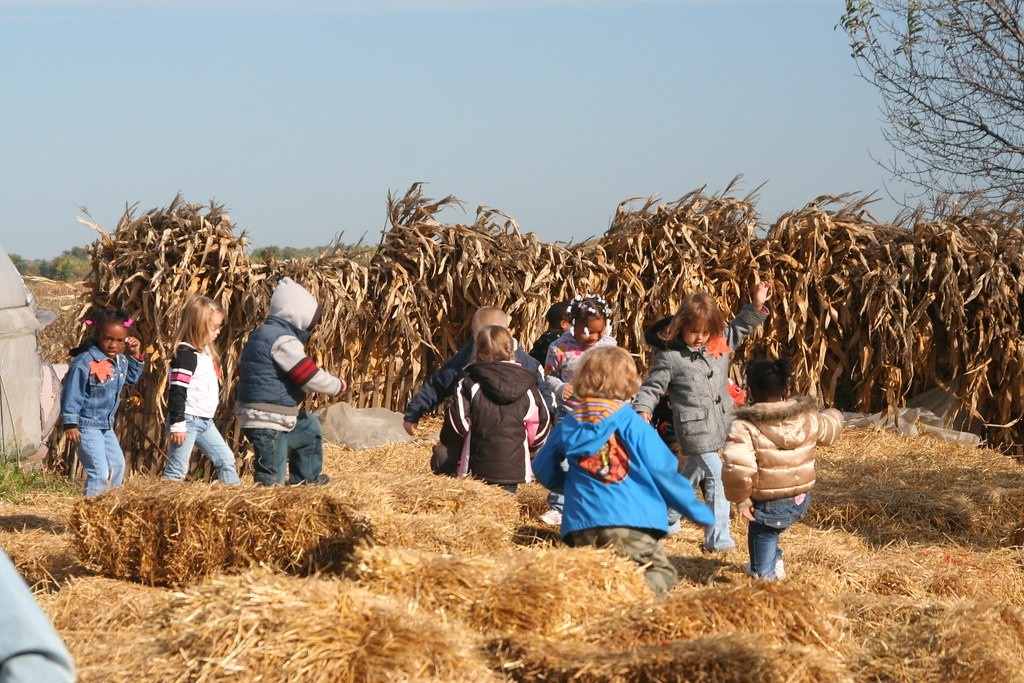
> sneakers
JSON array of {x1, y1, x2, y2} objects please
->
[{"x1": 538, "y1": 509, "x2": 562, "y2": 527}]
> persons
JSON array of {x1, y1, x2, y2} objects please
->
[
  {"x1": 402, "y1": 279, "x2": 846, "y2": 598},
  {"x1": 60, "y1": 309, "x2": 145, "y2": 503},
  {"x1": 237, "y1": 277, "x2": 347, "y2": 490},
  {"x1": 160, "y1": 297, "x2": 242, "y2": 487}
]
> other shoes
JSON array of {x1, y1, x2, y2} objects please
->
[
  {"x1": 745, "y1": 562, "x2": 785, "y2": 582},
  {"x1": 314, "y1": 474, "x2": 328, "y2": 484}
]
